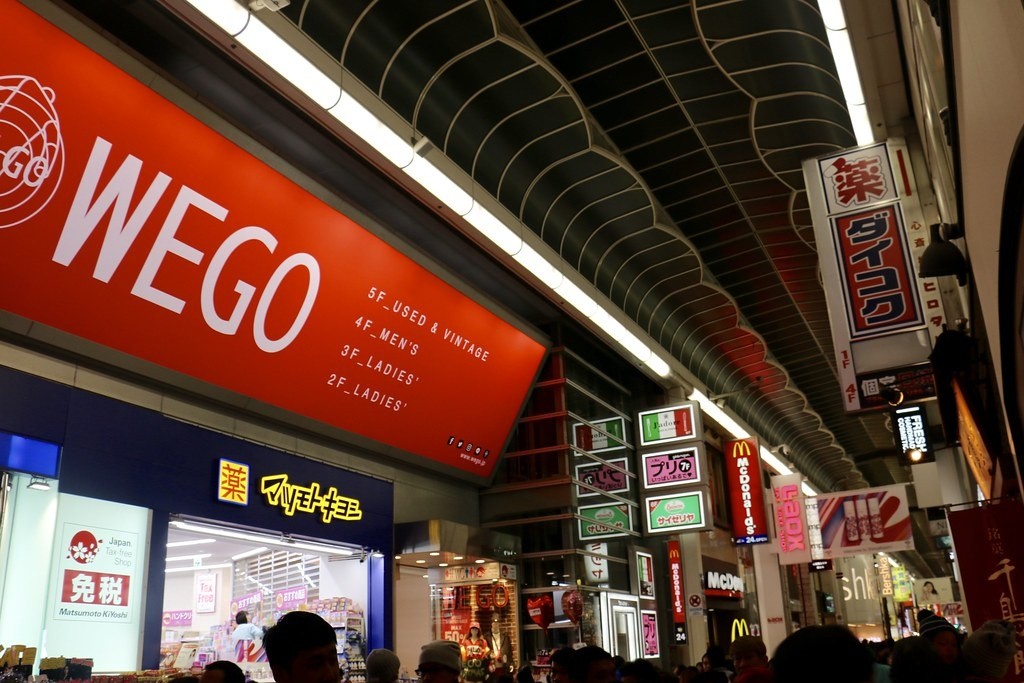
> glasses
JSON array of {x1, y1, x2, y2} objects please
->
[{"x1": 415, "y1": 666, "x2": 458, "y2": 676}]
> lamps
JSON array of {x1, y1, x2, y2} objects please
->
[
  {"x1": 686, "y1": 386, "x2": 819, "y2": 496},
  {"x1": 156, "y1": 1, "x2": 672, "y2": 384},
  {"x1": 915, "y1": 220, "x2": 967, "y2": 280},
  {"x1": 812, "y1": 0, "x2": 888, "y2": 148}
]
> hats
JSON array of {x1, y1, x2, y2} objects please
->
[
  {"x1": 419, "y1": 641, "x2": 460, "y2": 672},
  {"x1": 918, "y1": 609, "x2": 957, "y2": 645},
  {"x1": 366, "y1": 649, "x2": 400, "y2": 678},
  {"x1": 961, "y1": 619, "x2": 1017, "y2": 679}
]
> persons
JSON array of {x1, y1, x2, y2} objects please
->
[
  {"x1": 167, "y1": 610, "x2": 1024, "y2": 683},
  {"x1": 487, "y1": 622, "x2": 514, "y2": 673},
  {"x1": 460, "y1": 622, "x2": 491, "y2": 667},
  {"x1": 918, "y1": 581, "x2": 941, "y2": 604}
]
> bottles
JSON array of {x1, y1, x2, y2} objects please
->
[
  {"x1": 161, "y1": 648, "x2": 178, "y2": 656},
  {"x1": 349, "y1": 657, "x2": 366, "y2": 670},
  {"x1": 245, "y1": 668, "x2": 253, "y2": 681},
  {"x1": 253, "y1": 668, "x2": 272, "y2": 678},
  {"x1": 349, "y1": 671, "x2": 366, "y2": 683}
]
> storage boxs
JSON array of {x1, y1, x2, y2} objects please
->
[{"x1": 305, "y1": 596, "x2": 366, "y2": 683}]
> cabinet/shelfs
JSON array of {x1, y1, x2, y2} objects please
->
[{"x1": 332, "y1": 610, "x2": 364, "y2": 683}]
